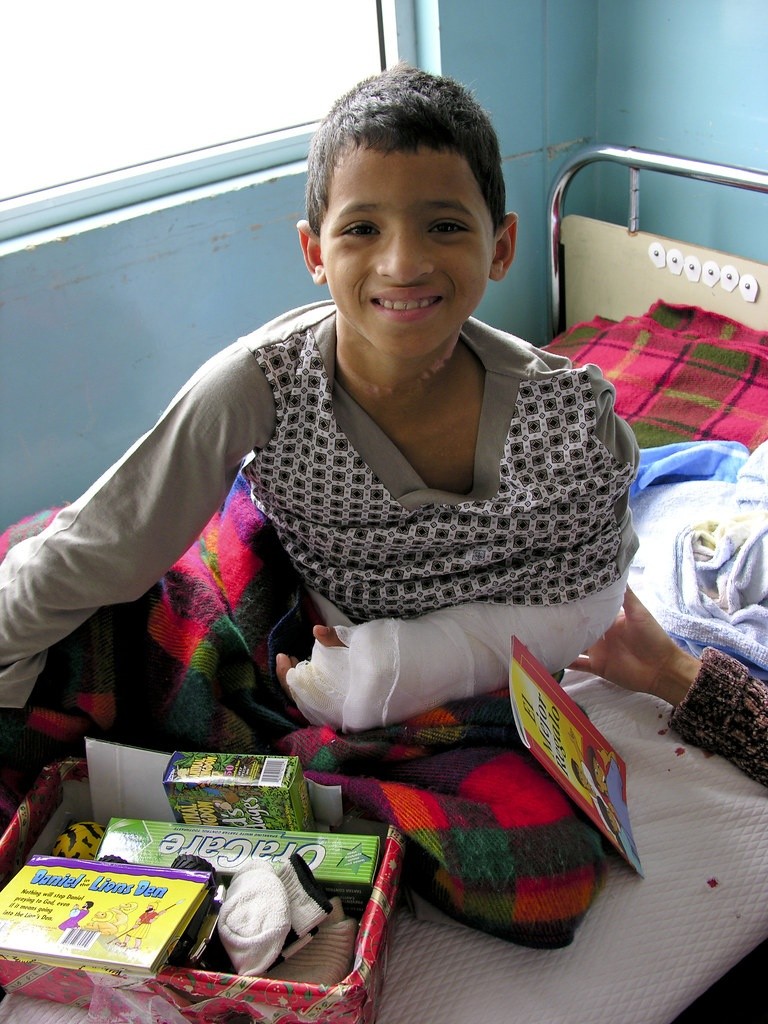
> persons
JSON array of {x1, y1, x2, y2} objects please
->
[
  {"x1": 0, "y1": 64, "x2": 642, "y2": 950},
  {"x1": 566, "y1": 578, "x2": 768, "y2": 789}
]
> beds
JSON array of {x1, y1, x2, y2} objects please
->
[{"x1": 0, "y1": 143, "x2": 768, "y2": 1024}]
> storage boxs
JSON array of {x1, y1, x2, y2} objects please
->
[
  {"x1": 0, "y1": 754, "x2": 406, "y2": 1024},
  {"x1": 93, "y1": 816, "x2": 381, "y2": 916},
  {"x1": 161, "y1": 749, "x2": 317, "y2": 831}
]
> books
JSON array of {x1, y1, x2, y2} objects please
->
[
  {"x1": 509, "y1": 634, "x2": 645, "y2": 879},
  {"x1": 0, "y1": 853, "x2": 212, "y2": 979}
]
